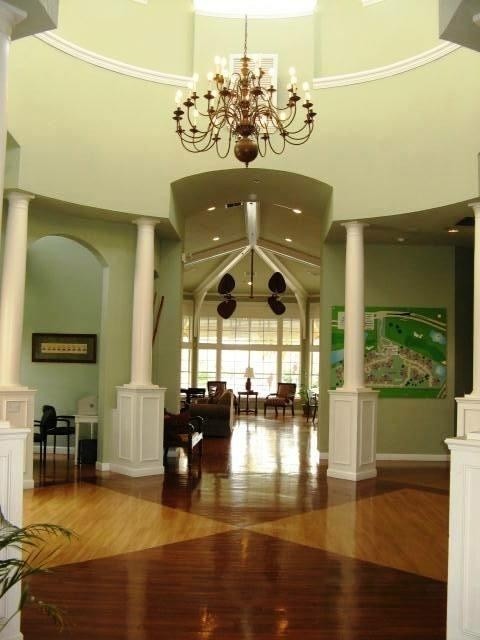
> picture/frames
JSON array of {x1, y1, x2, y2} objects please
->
[{"x1": 31, "y1": 332, "x2": 97, "y2": 363}]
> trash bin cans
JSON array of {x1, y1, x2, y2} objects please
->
[{"x1": 79, "y1": 439, "x2": 97, "y2": 465}]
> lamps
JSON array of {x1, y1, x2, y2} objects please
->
[
  {"x1": 217, "y1": 273, "x2": 237, "y2": 319},
  {"x1": 267, "y1": 272, "x2": 287, "y2": 315},
  {"x1": 172, "y1": 16, "x2": 316, "y2": 168},
  {"x1": 244, "y1": 368, "x2": 254, "y2": 392}
]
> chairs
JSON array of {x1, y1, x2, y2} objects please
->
[
  {"x1": 264, "y1": 383, "x2": 297, "y2": 416},
  {"x1": 163, "y1": 381, "x2": 237, "y2": 478},
  {"x1": 306, "y1": 388, "x2": 319, "y2": 422},
  {"x1": 34, "y1": 405, "x2": 75, "y2": 466}
]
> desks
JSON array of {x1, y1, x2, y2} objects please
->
[
  {"x1": 238, "y1": 392, "x2": 258, "y2": 416},
  {"x1": 71, "y1": 415, "x2": 98, "y2": 465}
]
